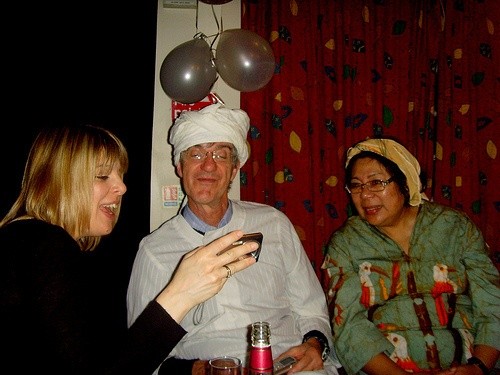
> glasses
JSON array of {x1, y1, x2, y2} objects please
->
[
  {"x1": 181, "y1": 148, "x2": 234, "y2": 163},
  {"x1": 345, "y1": 176, "x2": 395, "y2": 193}
]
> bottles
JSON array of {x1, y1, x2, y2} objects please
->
[{"x1": 250, "y1": 321, "x2": 274, "y2": 375}]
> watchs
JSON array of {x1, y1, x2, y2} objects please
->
[
  {"x1": 302, "y1": 330, "x2": 330, "y2": 362},
  {"x1": 468, "y1": 356, "x2": 489, "y2": 375}
]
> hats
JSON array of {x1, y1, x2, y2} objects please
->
[{"x1": 169, "y1": 103, "x2": 251, "y2": 168}]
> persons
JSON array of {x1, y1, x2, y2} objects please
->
[
  {"x1": 127, "y1": 102, "x2": 342, "y2": 375},
  {"x1": 321, "y1": 138, "x2": 500, "y2": 375},
  {"x1": 0, "y1": 123, "x2": 259, "y2": 375}
]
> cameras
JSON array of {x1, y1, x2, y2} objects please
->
[{"x1": 215, "y1": 231, "x2": 263, "y2": 265}]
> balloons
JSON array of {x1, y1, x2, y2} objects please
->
[
  {"x1": 159, "y1": 28, "x2": 216, "y2": 103},
  {"x1": 215, "y1": 29, "x2": 276, "y2": 92}
]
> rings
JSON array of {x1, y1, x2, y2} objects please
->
[{"x1": 223, "y1": 265, "x2": 231, "y2": 278}]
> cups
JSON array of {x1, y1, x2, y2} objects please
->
[{"x1": 208, "y1": 356, "x2": 243, "y2": 375}]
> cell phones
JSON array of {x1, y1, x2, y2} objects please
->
[{"x1": 274, "y1": 356, "x2": 296, "y2": 375}]
